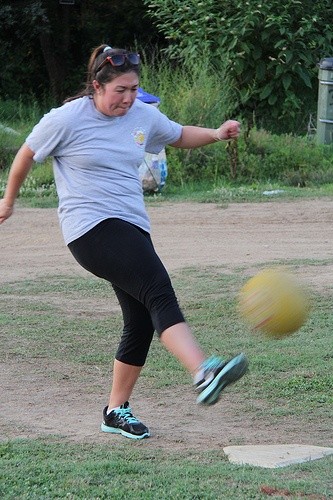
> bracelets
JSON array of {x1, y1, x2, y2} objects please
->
[
  {"x1": 1, "y1": 198, "x2": 14, "y2": 210},
  {"x1": 213, "y1": 128, "x2": 223, "y2": 142}
]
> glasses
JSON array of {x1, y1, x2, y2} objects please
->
[{"x1": 95, "y1": 52, "x2": 141, "y2": 75}]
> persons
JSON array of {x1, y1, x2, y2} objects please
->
[{"x1": 0, "y1": 43, "x2": 249, "y2": 440}]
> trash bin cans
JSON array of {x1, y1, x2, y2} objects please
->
[{"x1": 314, "y1": 58, "x2": 332, "y2": 148}]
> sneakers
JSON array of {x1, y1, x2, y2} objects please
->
[
  {"x1": 100, "y1": 401, "x2": 150, "y2": 439},
  {"x1": 192, "y1": 351, "x2": 249, "y2": 408}
]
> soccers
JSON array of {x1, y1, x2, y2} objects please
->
[{"x1": 240, "y1": 273, "x2": 310, "y2": 339}]
